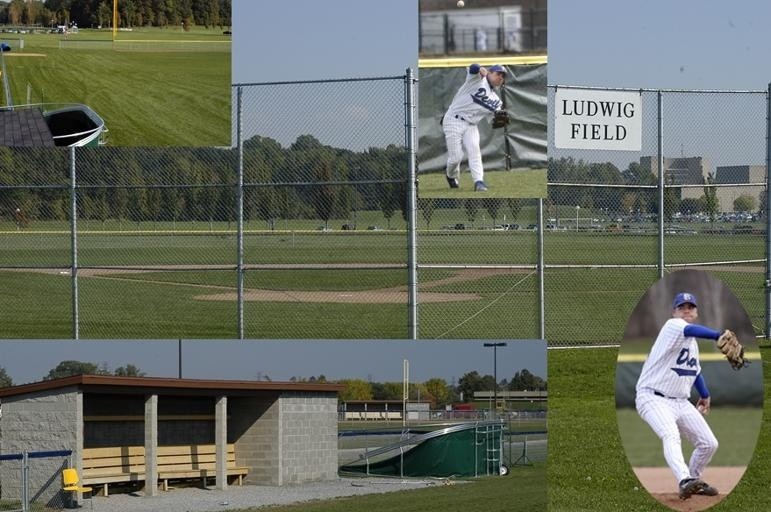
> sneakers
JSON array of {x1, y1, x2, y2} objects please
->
[
  {"x1": 691, "y1": 476, "x2": 720, "y2": 496},
  {"x1": 446, "y1": 172, "x2": 460, "y2": 188},
  {"x1": 474, "y1": 180, "x2": 489, "y2": 192},
  {"x1": 678, "y1": 477, "x2": 706, "y2": 501}
]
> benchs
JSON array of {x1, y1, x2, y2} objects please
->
[{"x1": 82, "y1": 444, "x2": 249, "y2": 496}]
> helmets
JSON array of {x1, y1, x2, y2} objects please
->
[{"x1": 486, "y1": 64, "x2": 510, "y2": 77}]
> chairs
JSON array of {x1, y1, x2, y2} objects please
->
[{"x1": 60, "y1": 468, "x2": 92, "y2": 511}]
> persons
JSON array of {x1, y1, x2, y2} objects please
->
[
  {"x1": 448, "y1": 23, "x2": 456, "y2": 51},
  {"x1": 632, "y1": 292, "x2": 752, "y2": 500},
  {"x1": 442, "y1": 62, "x2": 511, "y2": 192},
  {"x1": 506, "y1": 28, "x2": 521, "y2": 53},
  {"x1": 473, "y1": 27, "x2": 488, "y2": 53}
]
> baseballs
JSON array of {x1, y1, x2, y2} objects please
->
[
  {"x1": 457, "y1": 0, "x2": 465, "y2": 9},
  {"x1": 698, "y1": 405, "x2": 707, "y2": 413}
]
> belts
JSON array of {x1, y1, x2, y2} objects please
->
[
  {"x1": 455, "y1": 114, "x2": 477, "y2": 126},
  {"x1": 655, "y1": 389, "x2": 678, "y2": 399}
]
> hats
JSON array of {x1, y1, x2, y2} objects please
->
[{"x1": 672, "y1": 292, "x2": 699, "y2": 309}]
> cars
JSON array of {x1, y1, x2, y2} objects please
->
[{"x1": 318, "y1": 211, "x2": 758, "y2": 234}]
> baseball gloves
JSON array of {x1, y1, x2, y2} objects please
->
[
  {"x1": 716, "y1": 329, "x2": 752, "y2": 371},
  {"x1": 491, "y1": 109, "x2": 509, "y2": 128}
]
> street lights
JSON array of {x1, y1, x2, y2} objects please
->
[
  {"x1": 575, "y1": 205, "x2": 580, "y2": 232},
  {"x1": 484, "y1": 342, "x2": 507, "y2": 407},
  {"x1": 16, "y1": 208, "x2": 20, "y2": 230}
]
